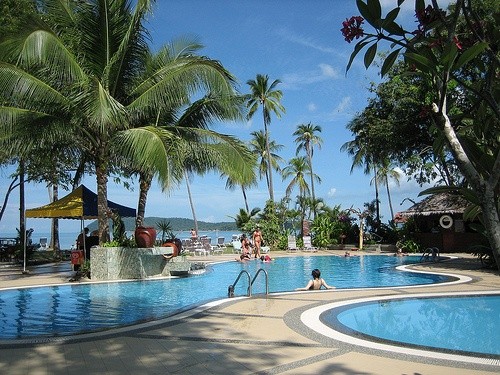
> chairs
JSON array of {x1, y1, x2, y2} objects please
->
[
  {"x1": 303, "y1": 235, "x2": 317, "y2": 252},
  {"x1": 180, "y1": 235, "x2": 271, "y2": 257},
  {"x1": 40, "y1": 238, "x2": 47, "y2": 249},
  {"x1": 288, "y1": 236, "x2": 298, "y2": 252}
]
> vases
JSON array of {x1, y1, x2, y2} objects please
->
[
  {"x1": 136, "y1": 227, "x2": 156, "y2": 247},
  {"x1": 163, "y1": 243, "x2": 179, "y2": 257}
]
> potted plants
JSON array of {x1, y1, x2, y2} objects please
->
[{"x1": 166, "y1": 233, "x2": 183, "y2": 255}]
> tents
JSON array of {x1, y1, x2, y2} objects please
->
[{"x1": 22, "y1": 184, "x2": 137, "y2": 271}]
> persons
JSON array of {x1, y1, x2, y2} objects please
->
[
  {"x1": 293, "y1": 268, "x2": 336, "y2": 291},
  {"x1": 190, "y1": 228, "x2": 195, "y2": 236},
  {"x1": 235, "y1": 227, "x2": 273, "y2": 263},
  {"x1": 394, "y1": 248, "x2": 409, "y2": 256},
  {"x1": 71, "y1": 228, "x2": 90, "y2": 250},
  {"x1": 345, "y1": 251, "x2": 352, "y2": 257}
]
